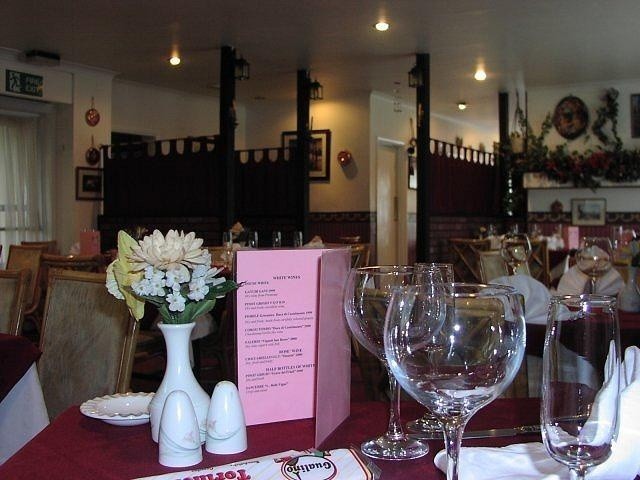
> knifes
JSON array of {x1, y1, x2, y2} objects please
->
[{"x1": 403, "y1": 424, "x2": 541, "y2": 440}]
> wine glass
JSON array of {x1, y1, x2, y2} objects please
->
[
  {"x1": 343, "y1": 265, "x2": 445, "y2": 460},
  {"x1": 401, "y1": 262, "x2": 456, "y2": 436},
  {"x1": 501, "y1": 234, "x2": 532, "y2": 275},
  {"x1": 247, "y1": 231, "x2": 258, "y2": 249},
  {"x1": 223, "y1": 232, "x2": 234, "y2": 266},
  {"x1": 294, "y1": 233, "x2": 303, "y2": 249},
  {"x1": 384, "y1": 283, "x2": 527, "y2": 480},
  {"x1": 273, "y1": 231, "x2": 281, "y2": 248},
  {"x1": 541, "y1": 295, "x2": 621, "y2": 480},
  {"x1": 575, "y1": 238, "x2": 612, "y2": 296}
]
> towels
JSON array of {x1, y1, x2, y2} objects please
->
[
  {"x1": 473, "y1": 276, "x2": 576, "y2": 323},
  {"x1": 434, "y1": 346, "x2": 640, "y2": 479},
  {"x1": 558, "y1": 265, "x2": 624, "y2": 298}
]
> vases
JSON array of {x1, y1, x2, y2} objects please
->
[{"x1": 149, "y1": 323, "x2": 212, "y2": 445}]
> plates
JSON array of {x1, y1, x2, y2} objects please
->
[{"x1": 81, "y1": 391, "x2": 156, "y2": 427}]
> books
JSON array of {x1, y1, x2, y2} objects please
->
[{"x1": 236, "y1": 248, "x2": 350, "y2": 446}]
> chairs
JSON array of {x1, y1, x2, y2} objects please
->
[
  {"x1": 475, "y1": 250, "x2": 509, "y2": 283},
  {"x1": 20, "y1": 241, "x2": 60, "y2": 255},
  {"x1": 6, "y1": 245, "x2": 46, "y2": 341},
  {"x1": 504, "y1": 240, "x2": 552, "y2": 292},
  {"x1": 41, "y1": 254, "x2": 100, "y2": 272},
  {"x1": 37, "y1": 270, "x2": 139, "y2": 422},
  {"x1": 1, "y1": 269, "x2": 32, "y2": 336},
  {"x1": 353, "y1": 286, "x2": 439, "y2": 406},
  {"x1": 447, "y1": 236, "x2": 491, "y2": 286},
  {"x1": 416, "y1": 295, "x2": 531, "y2": 397}
]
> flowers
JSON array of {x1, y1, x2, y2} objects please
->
[
  {"x1": 543, "y1": 143, "x2": 639, "y2": 186},
  {"x1": 105, "y1": 227, "x2": 249, "y2": 325}
]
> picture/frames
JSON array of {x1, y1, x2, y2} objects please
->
[
  {"x1": 571, "y1": 198, "x2": 607, "y2": 226},
  {"x1": 75, "y1": 166, "x2": 105, "y2": 201},
  {"x1": 281, "y1": 130, "x2": 331, "y2": 182}
]
